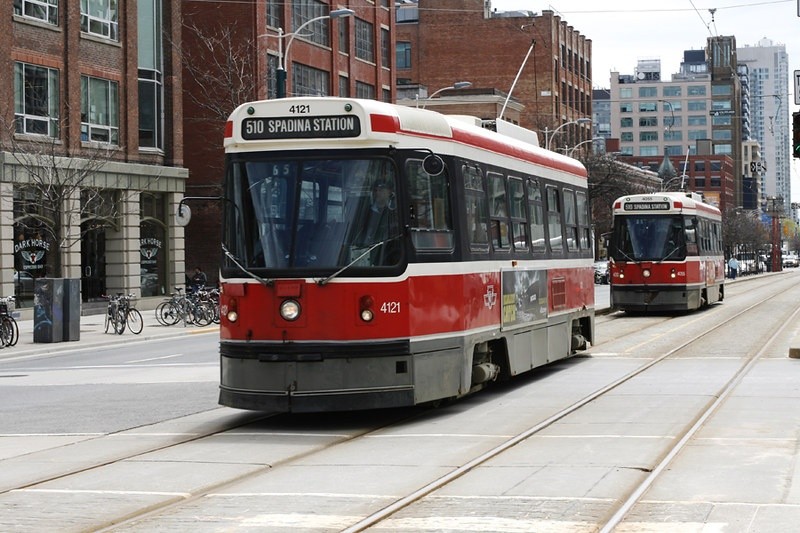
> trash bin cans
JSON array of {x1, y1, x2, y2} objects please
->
[
  {"x1": 63, "y1": 279, "x2": 81, "y2": 342},
  {"x1": 33, "y1": 278, "x2": 65, "y2": 343}
]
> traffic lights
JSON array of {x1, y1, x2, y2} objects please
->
[{"x1": 792, "y1": 112, "x2": 800, "y2": 158}]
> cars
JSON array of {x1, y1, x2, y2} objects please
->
[
  {"x1": 14, "y1": 271, "x2": 37, "y2": 295},
  {"x1": 723, "y1": 247, "x2": 799, "y2": 276}
]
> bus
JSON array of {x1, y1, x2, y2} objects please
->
[
  {"x1": 211, "y1": 94, "x2": 597, "y2": 415},
  {"x1": 600, "y1": 191, "x2": 726, "y2": 314}
]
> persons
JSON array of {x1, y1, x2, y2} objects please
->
[
  {"x1": 360, "y1": 179, "x2": 400, "y2": 266},
  {"x1": 729, "y1": 254, "x2": 740, "y2": 280},
  {"x1": 190, "y1": 267, "x2": 207, "y2": 294}
]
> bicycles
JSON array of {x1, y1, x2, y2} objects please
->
[
  {"x1": 0, "y1": 295, "x2": 20, "y2": 350},
  {"x1": 102, "y1": 292, "x2": 144, "y2": 335},
  {"x1": 155, "y1": 283, "x2": 223, "y2": 327}
]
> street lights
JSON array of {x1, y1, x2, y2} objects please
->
[
  {"x1": 413, "y1": 80, "x2": 474, "y2": 110},
  {"x1": 275, "y1": 8, "x2": 357, "y2": 97},
  {"x1": 564, "y1": 136, "x2": 606, "y2": 157},
  {"x1": 545, "y1": 117, "x2": 591, "y2": 151}
]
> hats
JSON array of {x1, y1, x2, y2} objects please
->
[{"x1": 370, "y1": 179, "x2": 393, "y2": 192}]
